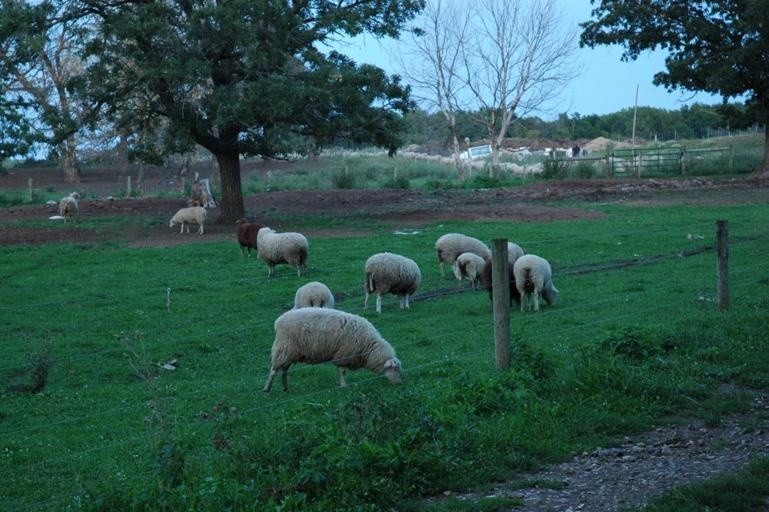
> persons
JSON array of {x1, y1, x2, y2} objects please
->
[
  {"x1": 572, "y1": 144, "x2": 580, "y2": 158},
  {"x1": 191, "y1": 184, "x2": 203, "y2": 207}
]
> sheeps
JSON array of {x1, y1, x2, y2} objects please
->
[
  {"x1": 236, "y1": 217, "x2": 266, "y2": 257},
  {"x1": 435, "y1": 232, "x2": 560, "y2": 314},
  {"x1": 59, "y1": 192, "x2": 80, "y2": 224},
  {"x1": 291, "y1": 281, "x2": 335, "y2": 309},
  {"x1": 169, "y1": 206, "x2": 208, "y2": 236},
  {"x1": 363, "y1": 252, "x2": 422, "y2": 314},
  {"x1": 256, "y1": 227, "x2": 309, "y2": 277},
  {"x1": 262, "y1": 307, "x2": 403, "y2": 393}
]
